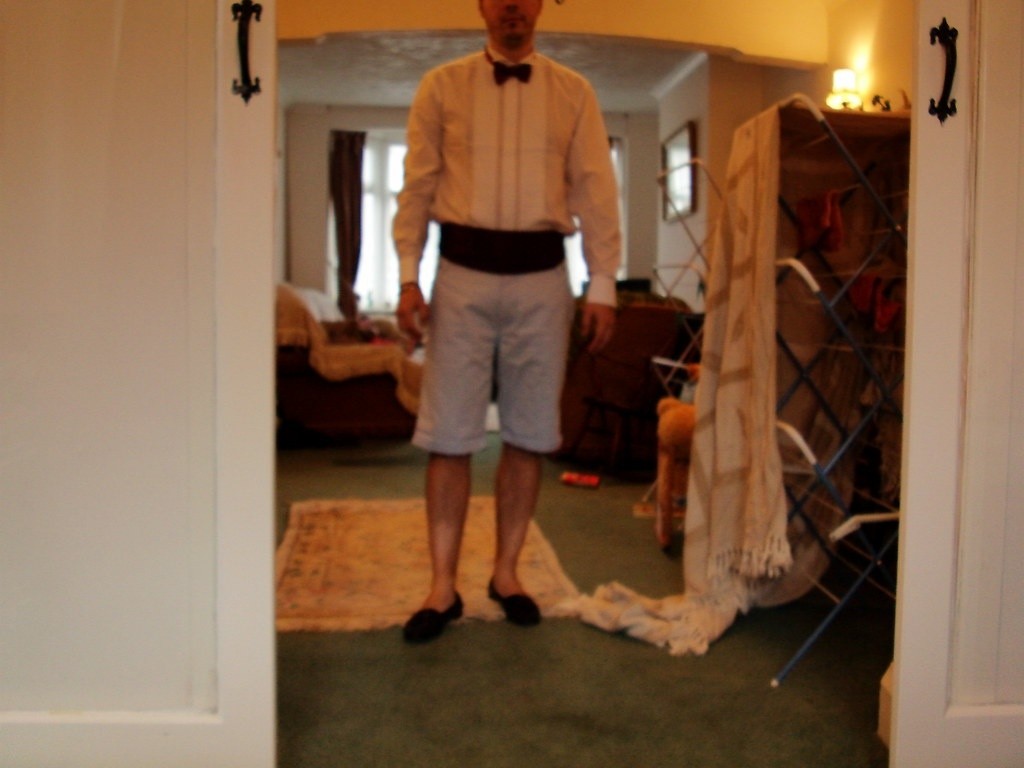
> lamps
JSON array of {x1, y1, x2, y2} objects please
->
[{"x1": 826, "y1": 68, "x2": 864, "y2": 110}]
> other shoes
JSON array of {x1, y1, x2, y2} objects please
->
[
  {"x1": 402, "y1": 591, "x2": 464, "y2": 644},
  {"x1": 487, "y1": 576, "x2": 542, "y2": 627}
]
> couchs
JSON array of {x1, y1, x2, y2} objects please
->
[
  {"x1": 554, "y1": 294, "x2": 701, "y2": 464},
  {"x1": 274, "y1": 287, "x2": 418, "y2": 440}
]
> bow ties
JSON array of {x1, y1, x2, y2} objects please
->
[{"x1": 484, "y1": 46, "x2": 533, "y2": 86}]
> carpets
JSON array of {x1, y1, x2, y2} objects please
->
[{"x1": 274, "y1": 495, "x2": 584, "y2": 633}]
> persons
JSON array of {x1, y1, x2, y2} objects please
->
[{"x1": 392, "y1": 0, "x2": 621, "y2": 645}]
